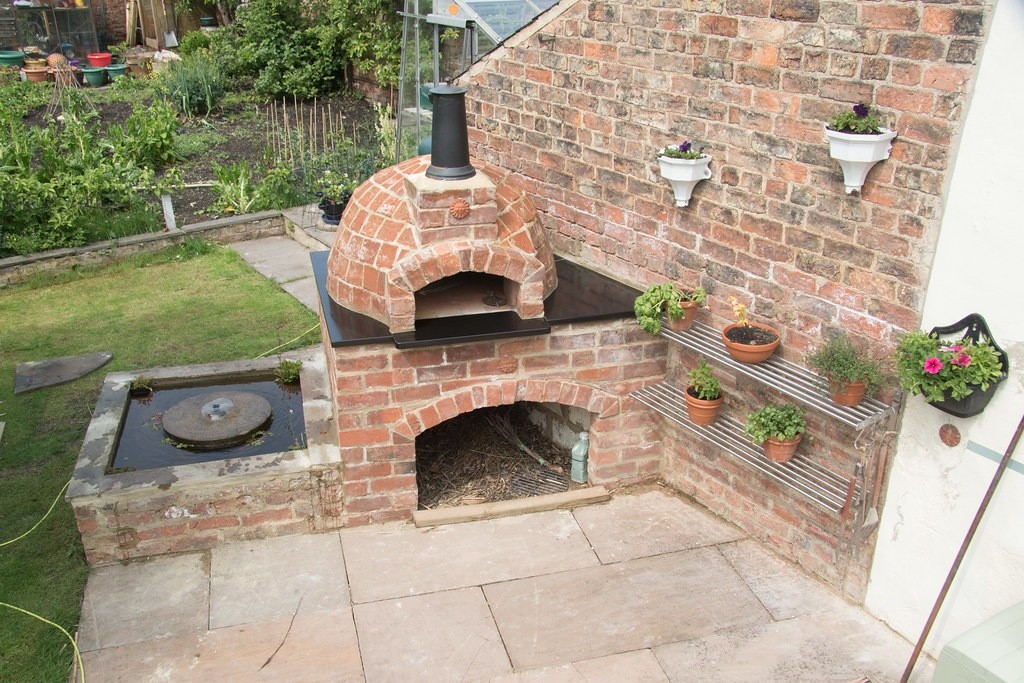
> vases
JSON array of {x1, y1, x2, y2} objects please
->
[
  {"x1": 321, "y1": 203, "x2": 345, "y2": 224},
  {"x1": 922, "y1": 311, "x2": 1007, "y2": 418},
  {"x1": 0, "y1": 50, "x2": 127, "y2": 87},
  {"x1": 659, "y1": 152, "x2": 713, "y2": 208},
  {"x1": 824, "y1": 123, "x2": 899, "y2": 193}
]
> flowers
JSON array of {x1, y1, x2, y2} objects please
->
[
  {"x1": 896, "y1": 324, "x2": 1007, "y2": 404},
  {"x1": 826, "y1": 102, "x2": 889, "y2": 134},
  {"x1": 313, "y1": 179, "x2": 351, "y2": 208},
  {"x1": 656, "y1": 140, "x2": 706, "y2": 159}
]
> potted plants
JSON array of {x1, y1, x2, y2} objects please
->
[
  {"x1": 743, "y1": 399, "x2": 813, "y2": 462},
  {"x1": 721, "y1": 296, "x2": 781, "y2": 364},
  {"x1": 807, "y1": 329, "x2": 893, "y2": 406},
  {"x1": 685, "y1": 360, "x2": 723, "y2": 425},
  {"x1": 634, "y1": 282, "x2": 708, "y2": 336}
]
